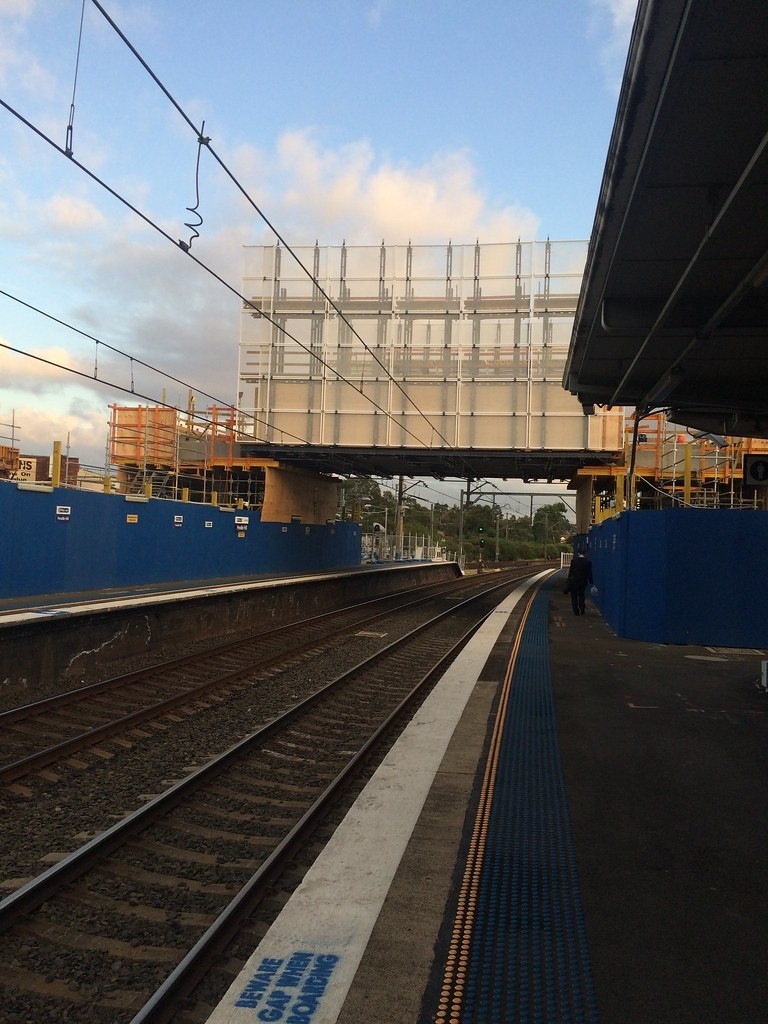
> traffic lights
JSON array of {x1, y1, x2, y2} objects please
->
[
  {"x1": 481, "y1": 539, "x2": 485, "y2": 548},
  {"x1": 479, "y1": 526, "x2": 482, "y2": 532}
]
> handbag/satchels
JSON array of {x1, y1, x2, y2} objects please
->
[
  {"x1": 561, "y1": 578, "x2": 571, "y2": 595},
  {"x1": 591, "y1": 585, "x2": 598, "y2": 597}
]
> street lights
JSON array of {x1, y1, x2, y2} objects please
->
[
  {"x1": 495, "y1": 521, "x2": 521, "y2": 563},
  {"x1": 397, "y1": 480, "x2": 429, "y2": 560},
  {"x1": 341, "y1": 497, "x2": 370, "y2": 522},
  {"x1": 366, "y1": 504, "x2": 409, "y2": 552}
]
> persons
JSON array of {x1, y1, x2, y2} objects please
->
[{"x1": 568, "y1": 547, "x2": 593, "y2": 616}]
